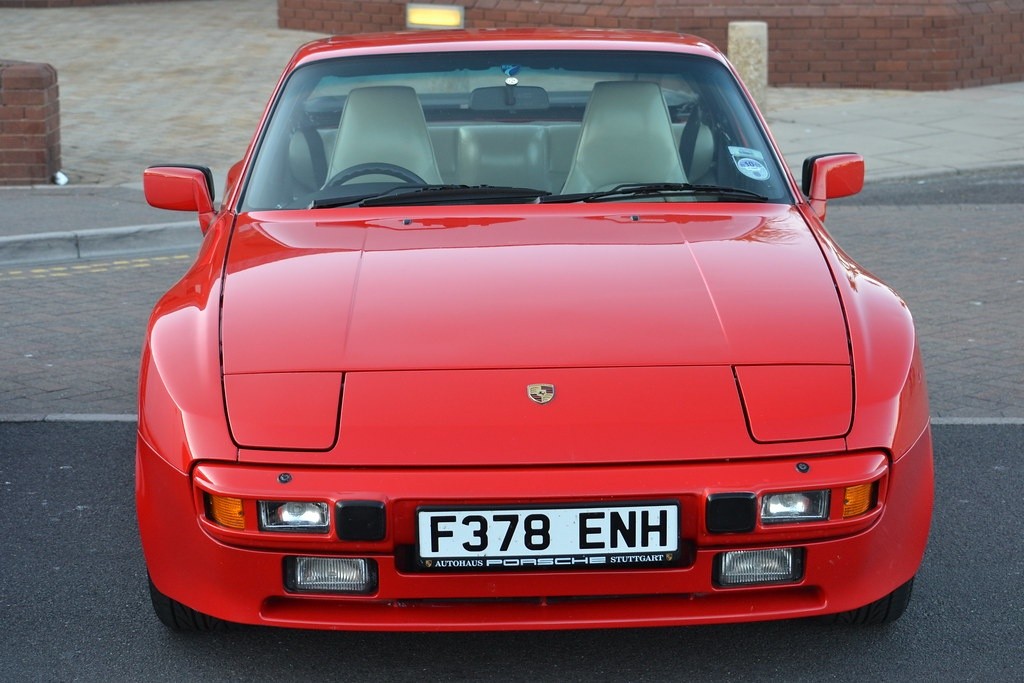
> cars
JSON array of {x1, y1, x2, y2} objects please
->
[{"x1": 135, "y1": 27, "x2": 935, "y2": 633}]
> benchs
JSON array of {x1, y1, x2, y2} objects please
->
[{"x1": 287, "y1": 120, "x2": 718, "y2": 200}]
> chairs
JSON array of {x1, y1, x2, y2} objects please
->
[
  {"x1": 560, "y1": 81, "x2": 695, "y2": 201},
  {"x1": 321, "y1": 86, "x2": 442, "y2": 188}
]
